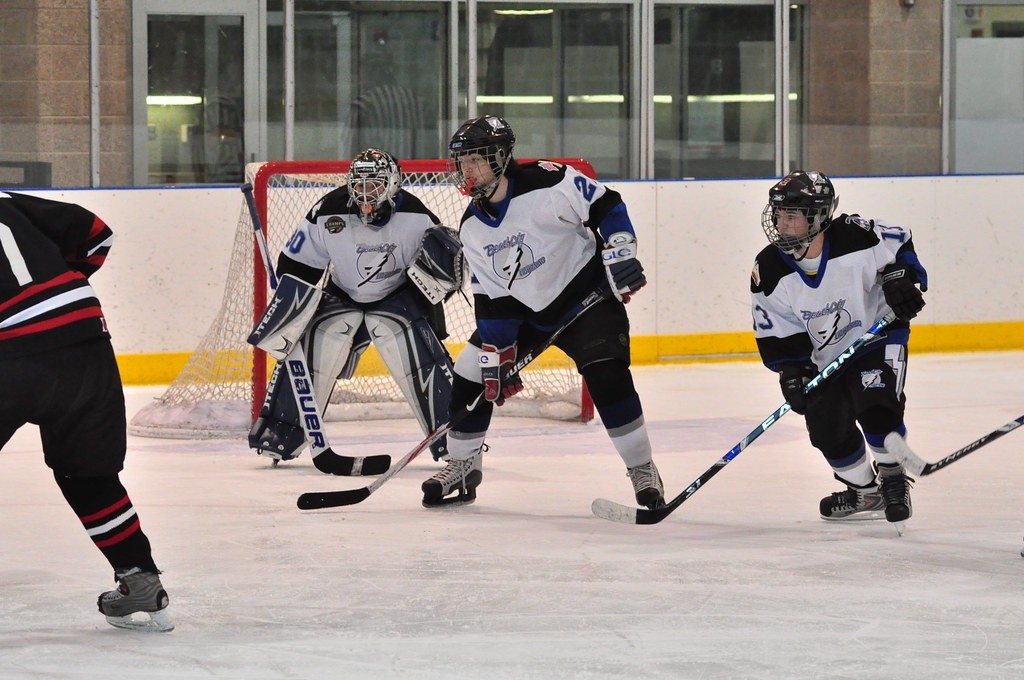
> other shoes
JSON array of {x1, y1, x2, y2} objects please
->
[
  {"x1": 430, "y1": 433, "x2": 453, "y2": 463},
  {"x1": 248, "y1": 418, "x2": 307, "y2": 467}
]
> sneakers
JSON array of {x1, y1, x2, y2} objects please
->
[
  {"x1": 625, "y1": 460, "x2": 667, "y2": 511},
  {"x1": 873, "y1": 460, "x2": 916, "y2": 537},
  {"x1": 421, "y1": 438, "x2": 489, "y2": 507},
  {"x1": 819, "y1": 473, "x2": 886, "y2": 521},
  {"x1": 96, "y1": 567, "x2": 176, "y2": 633}
]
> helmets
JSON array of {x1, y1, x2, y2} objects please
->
[
  {"x1": 449, "y1": 115, "x2": 516, "y2": 200},
  {"x1": 769, "y1": 170, "x2": 836, "y2": 255},
  {"x1": 347, "y1": 147, "x2": 402, "y2": 227}
]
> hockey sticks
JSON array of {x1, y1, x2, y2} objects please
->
[
  {"x1": 590, "y1": 286, "x2": 924, "y2": 526},
  {"x1": 295, "y1": 286, "x2": 608, "y2": 510},
  {"x1": 239, "y1": 181, "x2": 392, "y2": 478},
  {"x1": 881, "y1": 414, "x2": 1024, "y2": 479}
]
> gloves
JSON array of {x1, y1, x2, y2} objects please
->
[
  {"x1": 780, "y1": 362, "x2": 824, "y2": 415},
  {"x1": 602, "y1": 237, "x2": 647, "y2": 304},
  {"x1": 476, "y1": 340, "x2": 525, "y2": 407},
  {"x1": 881, "y1": 262, "x2": 927, "y2": 324}
]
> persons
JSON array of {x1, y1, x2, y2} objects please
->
[
  {"x1": 246, "y1": 146, "x2": 489, "y2": 466},
  {"x1": 750, "y1": 170, "x2": 928, "y2": 537},
  {"x1": 421, "y1": 113, "x2": 665, "y2": 511},
  {"x1": 1, "y1": 190, "x2": 177, "y2": 634}
]
